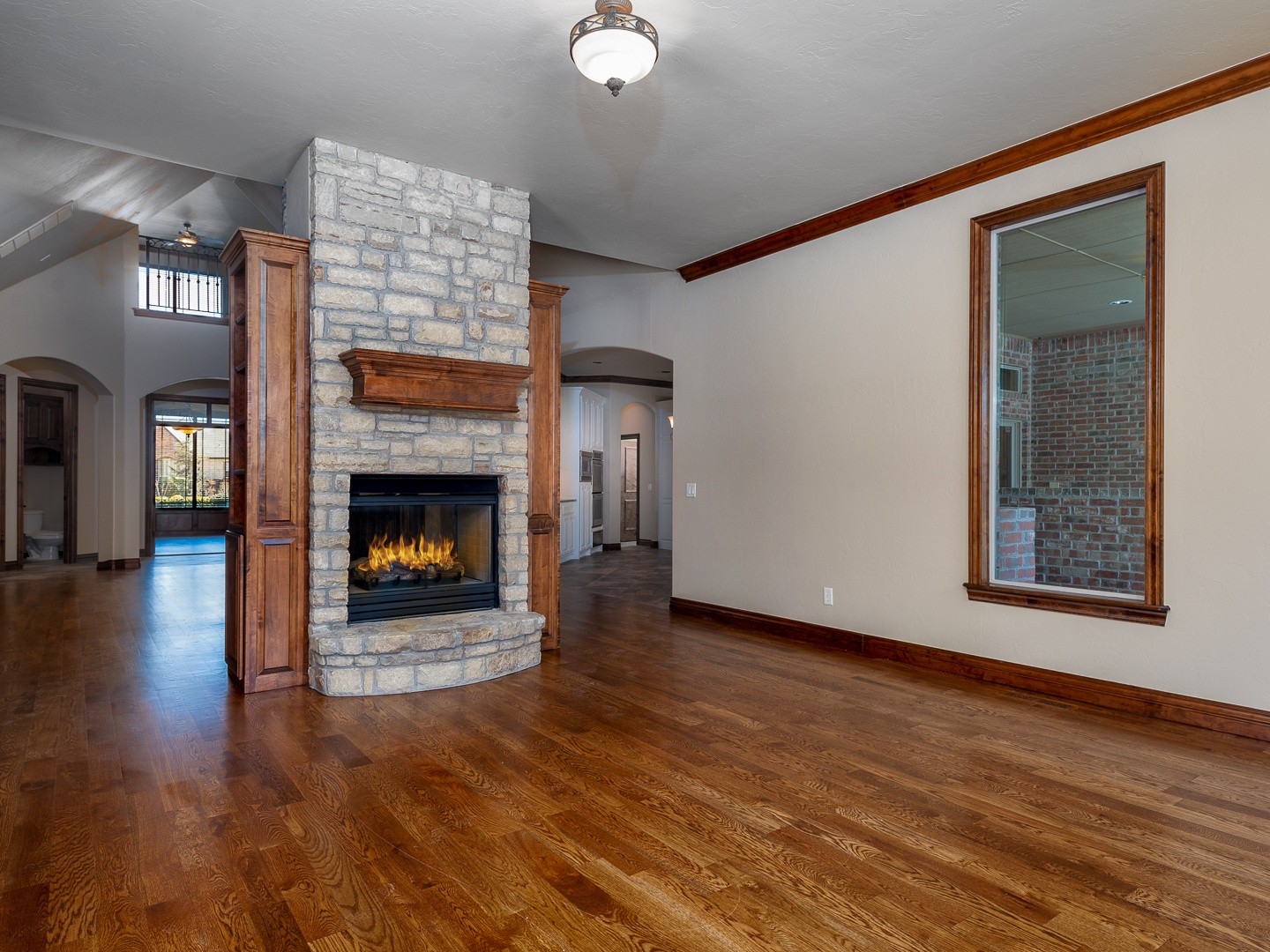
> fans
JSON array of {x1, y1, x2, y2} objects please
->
[{"x1": 147, "y1": 222, "x2": 226, "y2": 249}]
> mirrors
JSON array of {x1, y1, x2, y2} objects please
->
[{"x1": 963, "y1": 160, "x2": 1170, "y2": 629}]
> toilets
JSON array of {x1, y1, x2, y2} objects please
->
[{"x1": 23, "y1": 508, "x2": 64, "y2": 560}]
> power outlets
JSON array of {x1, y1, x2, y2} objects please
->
[{"x1": 823, "y1": 587, "x2": 833, "y2": 605}]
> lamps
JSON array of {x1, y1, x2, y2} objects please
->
[
  {"x1": 568, "y1": 0, "x2": 659, "y2": 97},
  {"x1": 178, "y1": 236, "x2": 198, "y2": 247},
  {"x1": 173, "y1": 403, "x2": 204, "y2": 438}
]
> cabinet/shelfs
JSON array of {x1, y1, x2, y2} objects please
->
[
  {"x1": 23, "y1": 392, "x2": 65, "y2": 466},
  {"x1": 528, "y1": 278, "x2": 571, "y2": 652},
  {"x1": 218, "y1": 226, "x2": 310, "y2": 694},
  {"x1": 559, "y1": 386, "x2": 607, "y2": 565}
]
